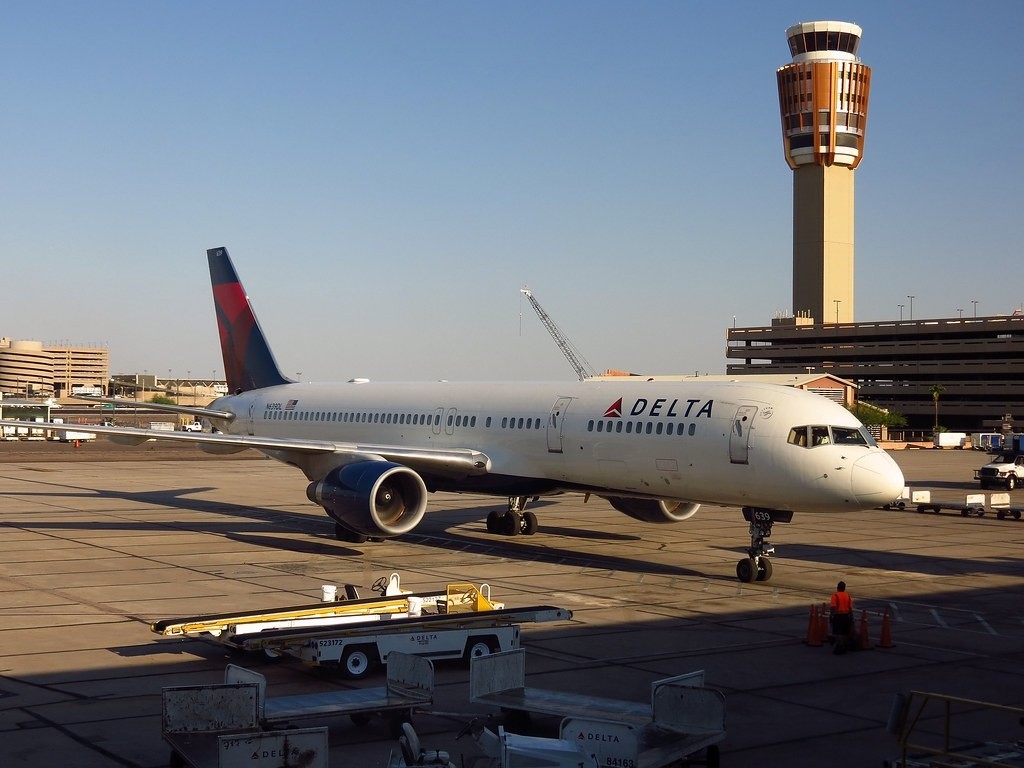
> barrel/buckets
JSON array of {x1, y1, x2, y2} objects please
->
[
  {"x1": 321, "y1": 584, "x2": 337, "y2": 601},
  {"x1": 408, "y1": 596, "x2": 421, "y2": 617}
]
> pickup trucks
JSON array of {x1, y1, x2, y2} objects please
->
[{"x1": 181, "y1": 421, "x2": 202, "y2": 433}]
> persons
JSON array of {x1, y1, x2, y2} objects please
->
[
  {"x1": 828, "y1": 581, "x2": 854, "y2": 654},
  {"x1": 818, "y1": 432, "x2": 831, "y2": 445}
]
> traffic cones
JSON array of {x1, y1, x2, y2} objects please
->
[
  {"x1": 802, "y1": 602, "x2": 831, "y2": 647},
  {"x1": 857, "y1": 609, "x2": 874, "y2": 651},
  {"x1": 875, "y1": 607, "x2": 896, "y2": 648},
  {"x1": 75, "y1": 439, "x2": 79, "y2": 448}
]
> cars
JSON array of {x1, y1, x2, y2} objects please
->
[{"x1": 50, "y1": 403, "x2": 63, "y2": 409}]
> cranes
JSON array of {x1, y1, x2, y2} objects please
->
[{"x1": 521, "y1": 284, "x2": 599, "y2": 380}]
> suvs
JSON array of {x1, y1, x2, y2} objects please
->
[{"x1": 979, "y1": 451, "x2": 1024, "y2": 490}]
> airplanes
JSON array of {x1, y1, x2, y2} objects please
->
[{"x1": 1, "y1": 243, "x2": 907, "y2": 585}]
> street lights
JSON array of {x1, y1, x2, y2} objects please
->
[
  {"x1": 971, "y1": 300, "x2": 979, "y2": 322},
  {"x1": 38, "y1": 377, "x2": 44, "y2": 398},
  {"x1": 110, "y1": 378, "x2": 115, "y2": 427},
  {"x1": 898, "y1": 304, "x2": 904, "y2": 326},
  {"x1": 833, "y1": 300, "x2": 842, "y2": 323},
  {"x1": 957, "y1": 308, "x2": 963, "y2": 318},
  {"x1": 907, "y1": 295, "x2": 915, "y2": 325}
]
